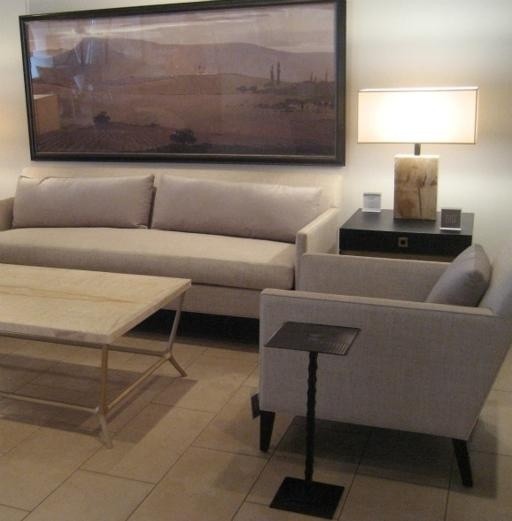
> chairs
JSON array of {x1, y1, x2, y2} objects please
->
[{"x1": 258, "y1": 250, "x2": 511, "y2": 488}]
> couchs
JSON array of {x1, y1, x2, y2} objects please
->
[{"x1": 0, "y1": 173, "x2": 359, "y2": 321}]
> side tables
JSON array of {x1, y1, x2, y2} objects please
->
[{"x1": 263, "y1": 319, "x2": 360, "y2": 520}]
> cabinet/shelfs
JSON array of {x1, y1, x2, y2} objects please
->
[{"x1": 339, "y1": 208, "x2": 474, "y2": 258}]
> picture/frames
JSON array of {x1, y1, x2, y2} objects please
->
[{"x1": 19, "y1": 0, "x2": 346, "y2": 166}]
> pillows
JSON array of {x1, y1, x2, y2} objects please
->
[{"x1": 423, "y1": 243, "x2": 492, "y2": 306}]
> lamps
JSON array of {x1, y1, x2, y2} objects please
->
[{"x1": 356, "y1": 87, "x2": 480, "y2": 222}]
